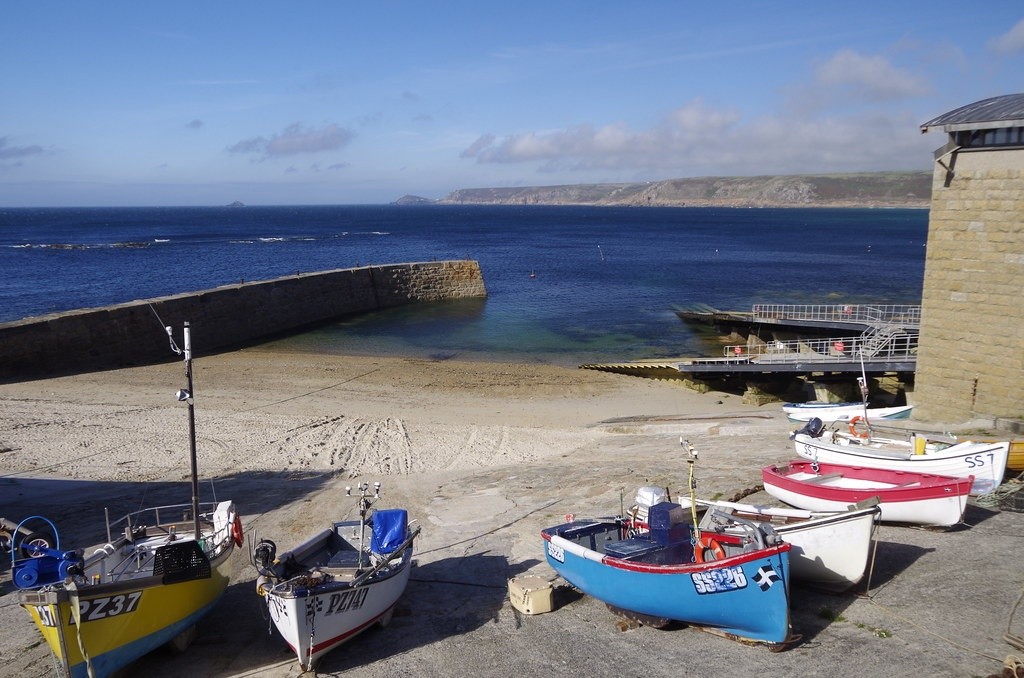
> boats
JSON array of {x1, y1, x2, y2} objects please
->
[
  {"x1": 155, "y1": 238, "x2": 171, "y2": 242},
  {"x1": 11, "y1": 301, "x2": 244, "y2": 678},
  {"x1": 782, "y1": 400, "x2": 913, "y2": 423},
  {"x1": 789, "y1": 344, "x2": 1024, "y2": 497},
  {"x1": 248, "y1": 482, "x2": 422, "y2": 672},
  {"x1": 626, "y1": 487, "x2": 881, "y2": 598},
  {"x1": 541, "y1": 437, "x2": 803, "y2": 653},
  {"x1": 763, "y1": 459, "x2": 975, "y2": 526}
]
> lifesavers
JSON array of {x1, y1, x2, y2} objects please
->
[
  {"x1": 693, "y1": 537, "x2": 726, "y2": 564},
  {"x1": 232, "y1": 514, "x2": 244, "y2": 549},
  {"x1": 849, "y1": 416, "x2": 871, "y2": 438}
]
